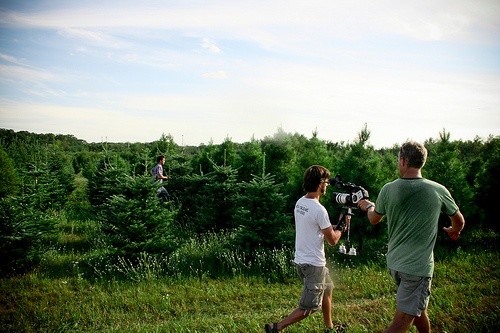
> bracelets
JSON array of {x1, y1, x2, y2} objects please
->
[{"x1": 366, "y1": 203, "x2": 374, "y2": 210}]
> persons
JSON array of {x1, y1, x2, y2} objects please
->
[
  {"x1": 150, "y1": 155, "x2": 170, "y2": 202},
  {"x1": 264, "y1": 166, "x2": 349, "y2": 333},
  {"x1": 357, "y1": 141, "x2": 465, "y2": 333}
]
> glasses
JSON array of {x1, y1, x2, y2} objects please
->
[{"x1": 325, "y1": 181, "x2": 330, "y2": 185}]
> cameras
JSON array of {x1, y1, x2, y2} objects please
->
[{"x1": 330, "y1": 174, "x2": 369, "y2": 208}]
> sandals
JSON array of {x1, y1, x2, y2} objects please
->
[
  {"x1": 265, "y1": 322, "x2": 282, "y2": 333},
  {"x1": 325, "y1": 324, "x2": 348, "y2": 333}
]
